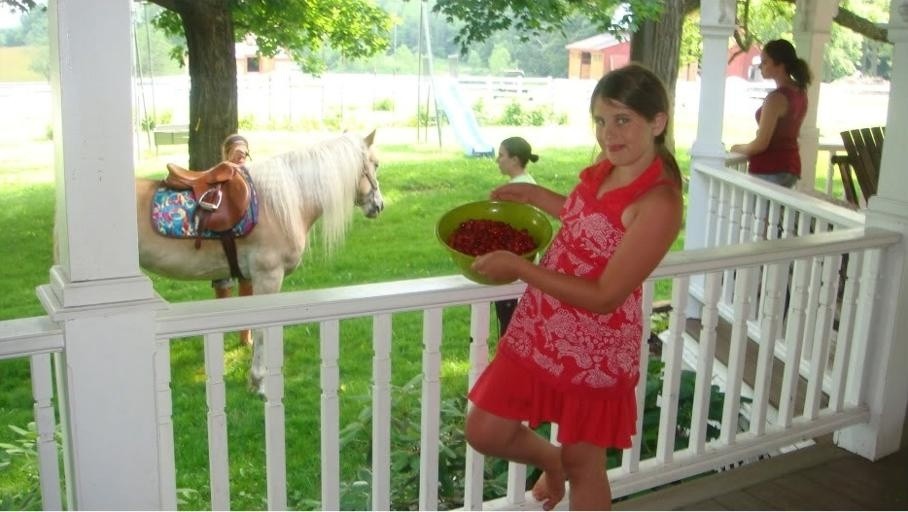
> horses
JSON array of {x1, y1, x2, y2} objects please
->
[{"x1": 53, "y1": 128, "x2": 385, "y2": 400}]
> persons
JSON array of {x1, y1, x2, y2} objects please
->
[
  {"x1": 490, "y1": 137, "x2": 539, "y2": 336},
  {"x1": 465, "y1": 63, "x2": 683, "y2": 510},
  {"x1": 728, "y1": 40, "x2": 815, "y2": 233},
  {"x1": 211, "y1": 135, "x2": 253, "y2": 347}
]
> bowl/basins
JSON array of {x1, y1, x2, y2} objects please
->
[{"x1": 435, "y1": 200, "x2": 554, "y2": 285}]
